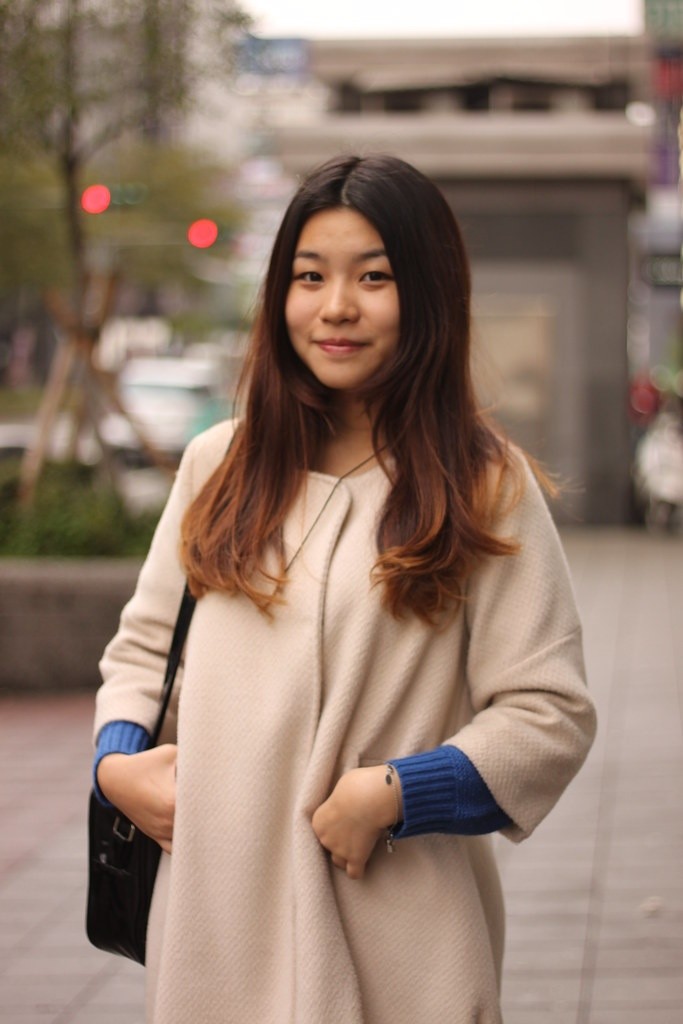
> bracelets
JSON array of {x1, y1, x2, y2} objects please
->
[{"x1": 384, "y1": 764, "x2": 399, "y2": 853}]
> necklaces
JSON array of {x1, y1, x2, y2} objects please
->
[{"x1": 284, "y1": 443, "x2": 388, "y2": 573}]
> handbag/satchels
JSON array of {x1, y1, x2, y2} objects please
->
[{"x1": 85, "y1": 788, "x2": 160, "y2": 964}]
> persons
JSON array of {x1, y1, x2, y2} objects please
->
[{"x1": 93, "y1": 154, "x2": 598, "y2": 1024}]
